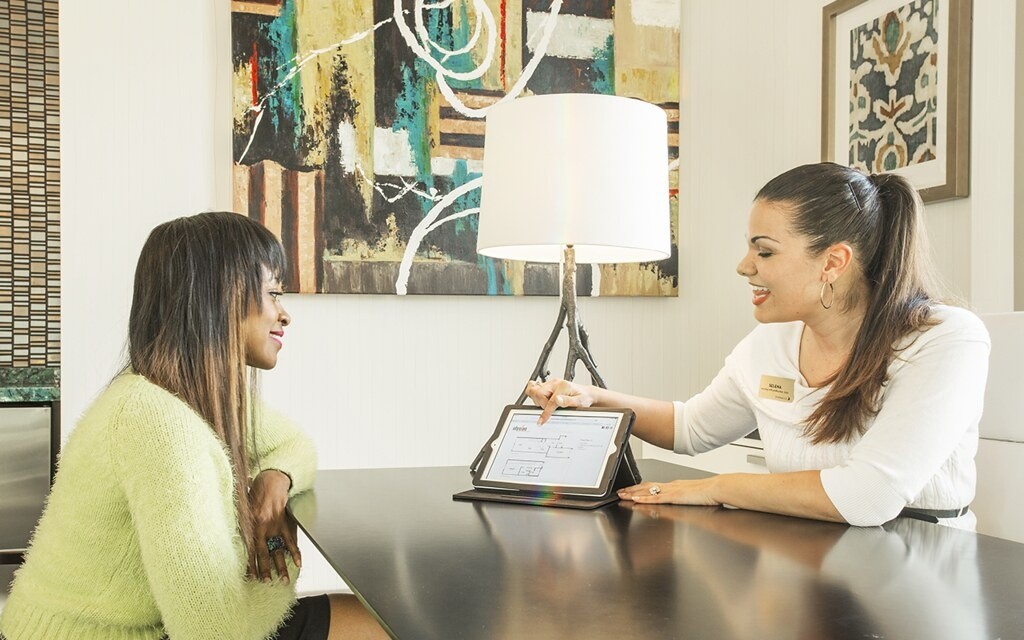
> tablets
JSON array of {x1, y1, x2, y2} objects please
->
[{"x1": 473, "y1": 405, "x2": 636, "y2": 501}]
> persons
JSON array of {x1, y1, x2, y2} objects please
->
[
  {"x1": 526, "y1": 161, "x2": 990, "y2": 535},
  {"x1": 0, "y1": 212, "x2": 392, "y2": 640}
]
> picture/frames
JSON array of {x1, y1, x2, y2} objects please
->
[{"x1": 821, "y1": 0, "x2": 974, "y2": 205}]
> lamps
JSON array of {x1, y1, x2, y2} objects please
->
[{"x1": 469, "y1": 93, "x2": 672, "y2": 494}]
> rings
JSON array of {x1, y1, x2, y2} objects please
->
[
  {"x1": 267, "y1": 535, "x2": 287, "y2": 557},
  {"x1": 651, "y1": 487, "x2": 660, "y2": 493}
]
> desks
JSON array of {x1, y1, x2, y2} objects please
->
[
  {"x1": 285, "y1": 457, "x2": 1024, "y2": 640},
  {"x1": 0, "y1": 367, "x2": 60, "y2": 565}
]
